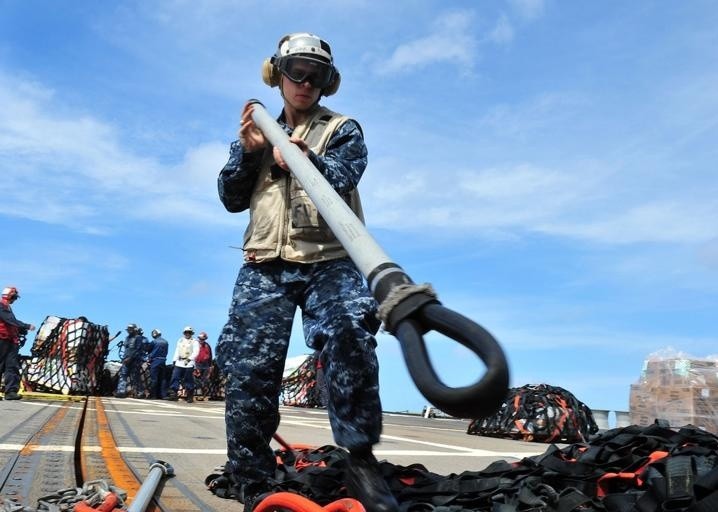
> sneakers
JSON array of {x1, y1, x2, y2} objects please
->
[
  {"x1": 5, "y1": 390, "x2": 22, "y2": 401},
  {"x1": 114, "y1": 390, "x2": 194, "y2": 402}
]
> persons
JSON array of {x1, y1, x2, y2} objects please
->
[
  {"x1": 214, "y1": 32, "x2": 382, "y2": 463},
  {"x1": 112, "y1": 324, "x2": 212, "y2": 403},
  {"x1": 1, "y1": 287, "x2": 36, "y2": 401}
]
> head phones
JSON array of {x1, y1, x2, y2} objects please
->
[{"x1": 261, "y1": 34, "x2": 341, "y2": 97}]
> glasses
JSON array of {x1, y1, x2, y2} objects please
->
[{"x1": 273, "y1": 54, "x2": 336, "y2": 90}]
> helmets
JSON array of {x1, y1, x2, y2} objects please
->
[
  {"x1": 125, "y1": 323, "x2": 209, "y2": 341},
  {"x1": 272, "y1": 31, "x2": 337, "y2": 97}
]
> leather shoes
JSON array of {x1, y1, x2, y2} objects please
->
[{"x1": 343, "y1": 446, "x2": 400, "y2": 511}]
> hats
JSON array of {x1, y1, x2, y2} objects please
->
[{"x1": 2, "y1": 286, "x2": 21, "y2": 300}]
